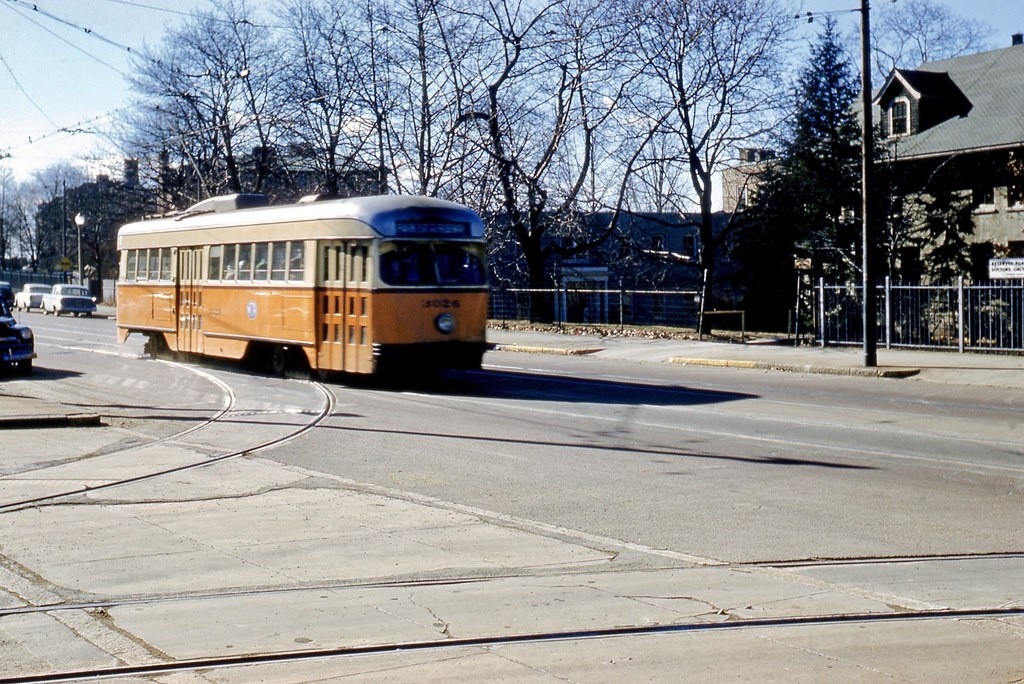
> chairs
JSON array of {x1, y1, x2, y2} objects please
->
[{"x1": 126, "y1": 260, "x2": 249, "y2": 279}]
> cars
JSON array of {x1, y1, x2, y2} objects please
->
[
  {"x1": 0, "y1": 281, "x2": 14, "y2": 307},
  {"x1": 40, "y1": 284, "x2": 98, "y2": 317},
  {"x1": 15, "y1": 283, "x2": 53, "y2": 312},
  {"x1": 0, "y1": 291, "x2": 37, "y2": 373}
]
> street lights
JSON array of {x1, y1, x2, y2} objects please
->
[{"x1": 75, "y1": 212, "x2": 85, "y2": 285}]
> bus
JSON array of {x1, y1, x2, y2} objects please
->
[{"x1": 116, "y1": 193, "x2": 490, "y2": 384}]
